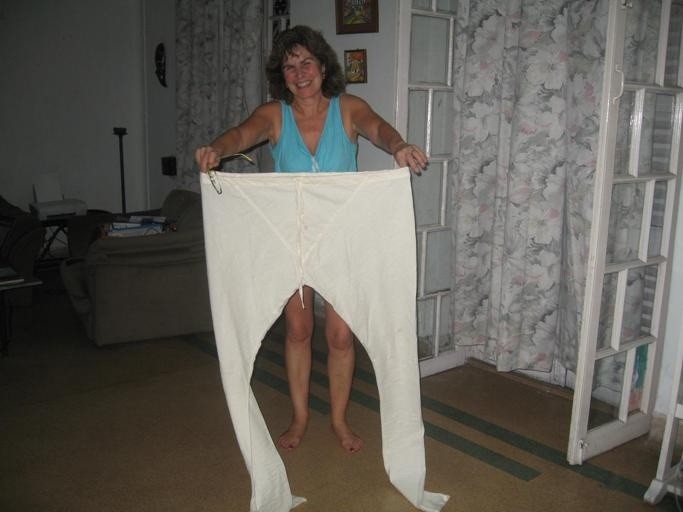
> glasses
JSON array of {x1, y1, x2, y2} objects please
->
[{"x1": 207, "y1": 152, "x2": 256, "y2": 197}]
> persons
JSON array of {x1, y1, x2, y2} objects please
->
[{"x1": 192, "y1": 23, "x2": 429, "y2": 455}]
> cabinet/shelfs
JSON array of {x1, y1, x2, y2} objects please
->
[{"x1": 33, "y1": 209, "x2": 112, "y2": 293}]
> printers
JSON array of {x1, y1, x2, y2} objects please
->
[{"x1": 29, "y1": 183, "x2": 87, "y2": 220}]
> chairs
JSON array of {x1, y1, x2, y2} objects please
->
[
  {"x1": 62, "y1": 188, "x2": 215, "y2": 347},
  {"x1": 0, "y1": 194, "x2": 44, "y2": 308}
]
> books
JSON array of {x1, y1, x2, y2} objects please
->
[{"x1": 104, "y1": 215, "x2": 173, "y2": 240}]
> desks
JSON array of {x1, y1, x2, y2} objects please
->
[{"x1": 0, "y1": 272, "x2": 43, "y2": 359}]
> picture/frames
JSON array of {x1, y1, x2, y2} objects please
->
[{"x1": 334, "y1": 0, "x2": 379, "y2": 84}]
